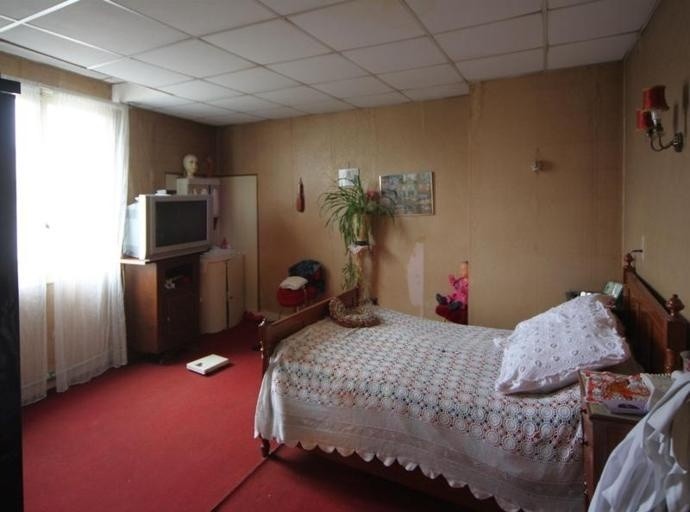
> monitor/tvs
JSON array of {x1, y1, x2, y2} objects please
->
[{"x1": 122, "y1": 195, "x2": 214, "y2": 261}]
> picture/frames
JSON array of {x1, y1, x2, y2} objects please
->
[{"x1": 378, "y1": 170, "x2": 434, "y2": 217}]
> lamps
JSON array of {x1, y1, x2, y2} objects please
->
[{"x1": 634, "y1": 83, "x2": 682, "y2": 152}]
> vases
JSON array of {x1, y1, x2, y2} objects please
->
[{"x1": 351, "y1": 215, "x2": 370, "y2": 245}]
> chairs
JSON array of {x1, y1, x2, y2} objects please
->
[{"x1": 276, "y1": 260, "x2": 324, "y2": 314}]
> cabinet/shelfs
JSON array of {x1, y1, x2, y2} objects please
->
[
  {"x1": 199, "y1": 248, "x2": 245, "y2": 335},
  {"x1": 120, "y1": 257, "x2": 199, "y2": 363}
]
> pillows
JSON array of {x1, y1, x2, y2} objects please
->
[{"x1": 494, "y1": 291, "x2": 632, "y2": 398}]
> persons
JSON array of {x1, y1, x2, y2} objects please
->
[
  {"x1": 182, "y1": 153, "x2": 199, "y2": 176},
  {"x1": 435, "y1": 260, "x2": 468, "y2": 311}
]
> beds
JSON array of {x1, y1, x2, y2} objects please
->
[{"x1": 256, "y1": 252, "x2": 689, "y2": 511}]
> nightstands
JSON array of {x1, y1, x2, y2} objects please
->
[{"x1": 580, "y1": 369, "x2": 670, "y2": 512}]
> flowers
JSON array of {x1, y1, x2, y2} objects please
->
[{"x1": 317, "y1": 175, "x2": 396, "y2": 215}]
